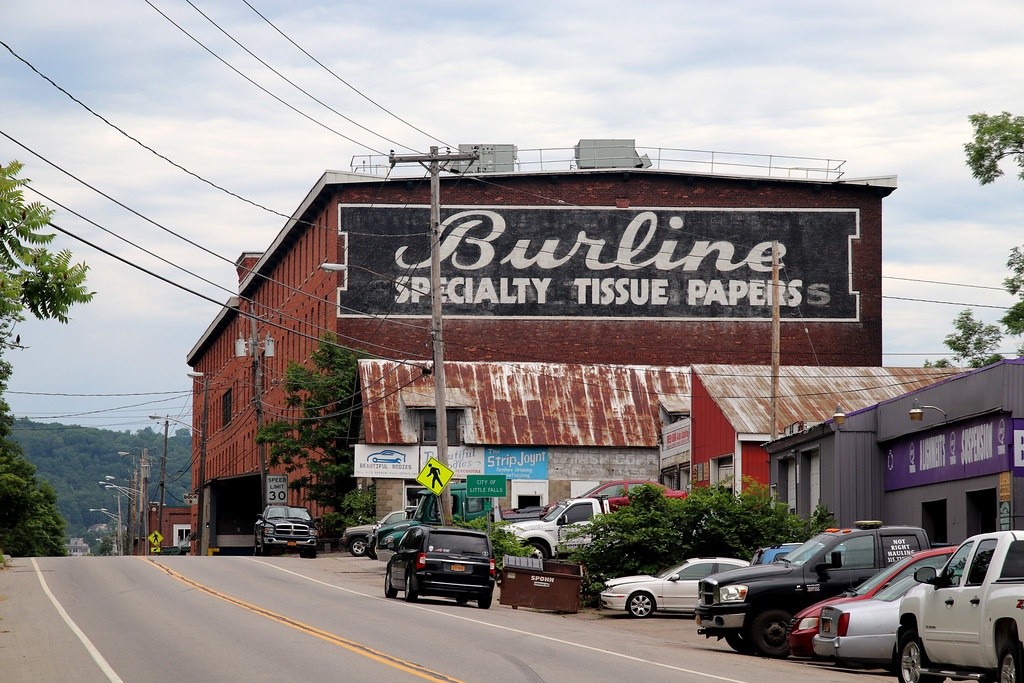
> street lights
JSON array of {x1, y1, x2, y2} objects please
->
[
  {"x1": 88, "y1": 452, "x2": 152, "y2": 556},
  {"x1": 148, "y1": 415, "x2": 208, "y2": 556},
  {"x1": 186, "y1": 372, "x2": 268, "y2": 512}
]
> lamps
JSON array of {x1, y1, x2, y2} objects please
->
[
  {"x1": 833, "y1": 403, "x2": 848, "y2": 430},
  {"x1": 909, "y1": 398, "x2": 947, "y2": 421}
]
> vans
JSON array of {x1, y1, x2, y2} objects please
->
[{"x1": 385, "y1": 525, "x2": 495, "y2": 609}]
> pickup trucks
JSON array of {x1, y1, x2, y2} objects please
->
[
  {"x1": 896, "y1": 531, "x2": 1024, "y2": 683},
  {"x1": 504, "y1": 498, "x2": 617, "y2": 559},
  {"x1": 377, "y1": 485, "x2": 541, "y2": 558},
  {"x1": 696, "y1": 521, "x2": 934, "y2": 658}
]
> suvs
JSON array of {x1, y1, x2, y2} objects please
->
[{"x1": 343, "y1": 506, "x2": 415, "y2": 557}]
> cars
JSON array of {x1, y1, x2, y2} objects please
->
[
  {"x1": 539, "y1": 479, "x2": 687, "y2": 519},
  {"x1": 255, "y1": 505, "x2": 319, "y2": 558},
  {"x1": 786, "y1": 546, "x2": 961, "y2": 675},
  {"x1": 601, "y1": 557, "x2": 750, "y2": 617}
]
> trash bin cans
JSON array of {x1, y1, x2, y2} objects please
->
[{"x1": 499, "y1": 553, "x2": 584, "y2": 615}]
